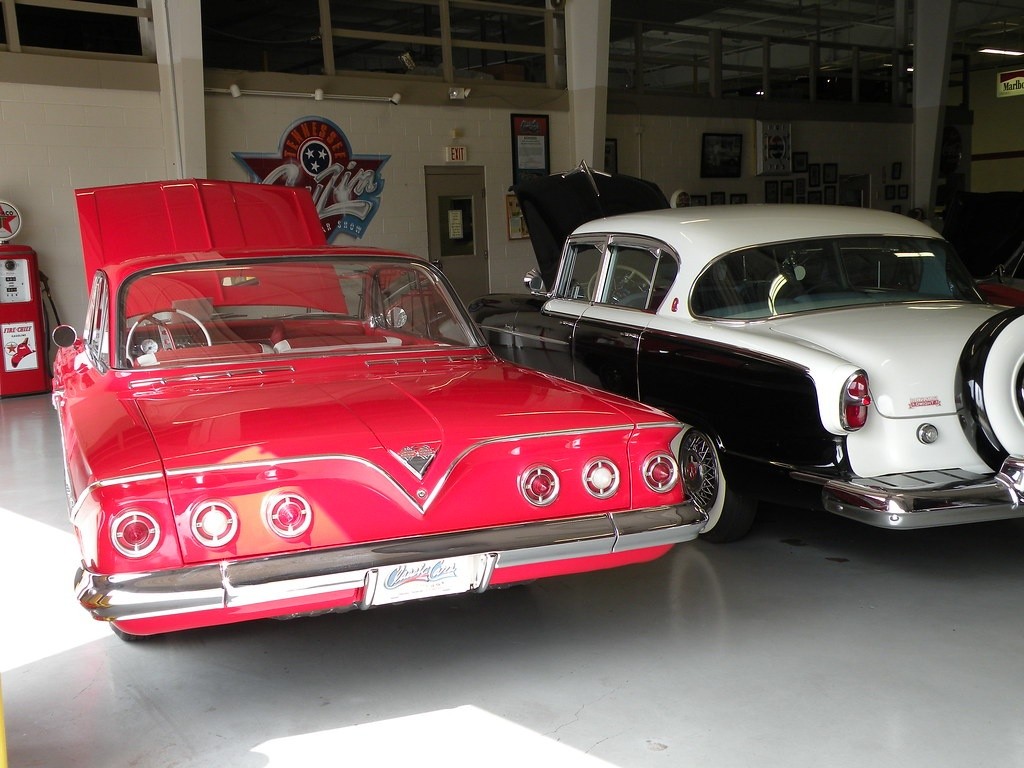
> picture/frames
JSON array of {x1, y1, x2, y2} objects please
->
[
  {"x1": 766, "y1": 151, "x2": 838, "y2": 205},
  {"x1": 711, "y1": 192, "x2": 725, "y2": 205},
  {"x1": 730, "y1": 194, "x2": 748, "y2": 204},
  {"x1": 690, "y1": 195, "x2": 706, "y2": 206},
  {"x1": 605, "y1": 138, "x2": 618, "y2": 174},
  {"x1": 898, "y1": 185, "x2": 909, "y2": 199},
  {"x1": 885, "y1": 184, "x2": 895, "y2": 200},
  {"x1": 506, "y1": 194, "x2": 531, "y2": 240},
  {"x1": 892, "y1": 205, "x2": 900, "y2": 214},
  {"x1": 510, "y1": 113, "x2": 549, "y2": 186},
  {"x1": 891, "y1": 162, "x2": 901, "y2": 180},
  {"x1": 701, "y1": 132, "x2": 742, "y2": 179}
]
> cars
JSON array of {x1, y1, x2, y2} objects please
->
[
  {"x1": 52, "y1": 178, "x2": 727, "y2": 645},
  {"x1": 464, "y1": 159, "x2": 1024, "y2": 546},
  {"x1": 970, "y1": 214, "x2": 1024, "y2": 305}
]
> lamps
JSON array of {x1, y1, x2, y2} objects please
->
[
  {"x1": 390, "y1": 93, "x2": 400, "y2": 105},
  {"x1": 314, "y1": 88, "x2": 325, "y2": 100},
  {"x1": 449, "y1": 87, "x2": 471, "y2": 99},
  {"x1": 229, "y1": 84, "x2": 241, "y2": 98}
]
